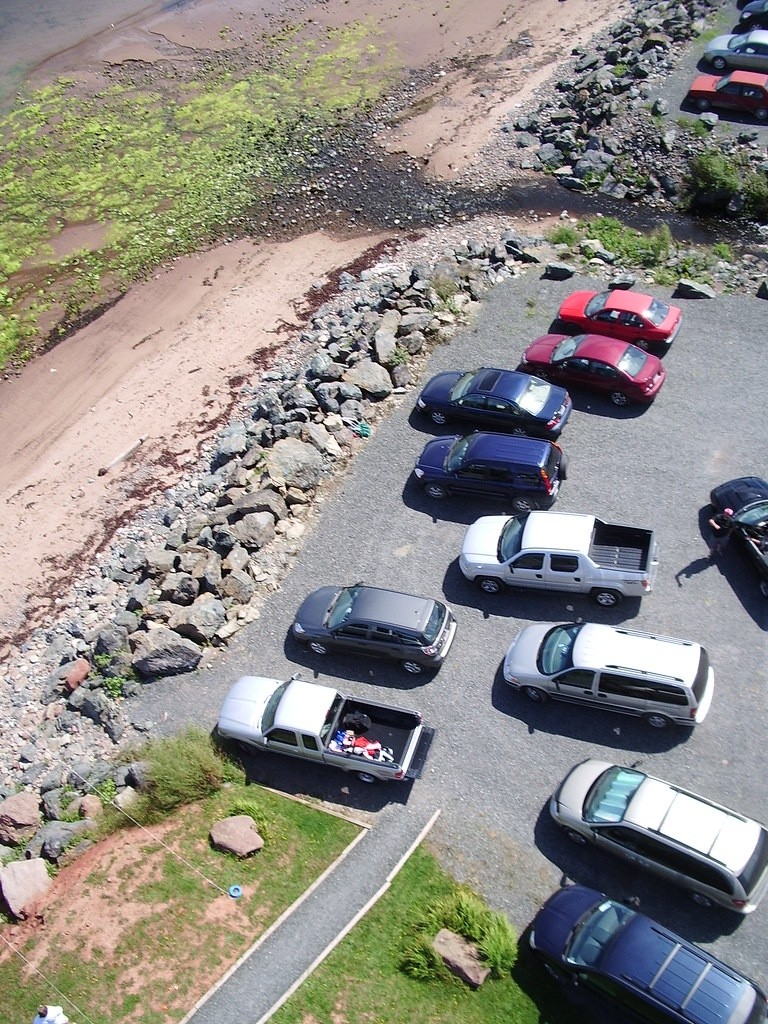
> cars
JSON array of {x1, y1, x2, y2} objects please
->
[
  {"x1": 701, "y1": 29, "x2": 768, "y2": 70},
  {"x1": 556, "y1": 288, "x2": 684, "y2": 351},
  {"x1": 687, "y1": 70, "x2": 768, "y2": 121},
  {"x1": 520, "y1": 334, "x2": 666, "y2": 407},
  {"x1": 710, "y1": 477, "x2": 768, "y2": 601},
  {"x1": 529, "y1": 884, "x2": 767, "y2": 1024},
  {"x1": 738, "y1": 0, "x2": 768, "y2": 33},
  {"x1": 416, "y1": 366, "x2": 573, "y2": 442}
]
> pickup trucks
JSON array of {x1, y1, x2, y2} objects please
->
[
  {"x1": 459, "y1": 511, "x2": 661, "y2": 607},
  {"x1": 216, "y1": 674, "x2": 435, "y2": 785}
]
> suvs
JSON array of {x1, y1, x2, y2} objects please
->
[
  {"x1": 411, "y1": 430, "x2": 570, "y2": 511},
  {"x1": 550, "y1": 757, "x2": 768, "y2": 913},
  {"x1": 293, "y1": 580, "x2": 459, "y2": 675},
  {"x1": 503, "y1": 617, "x2": 715, "y2": 730}
]
